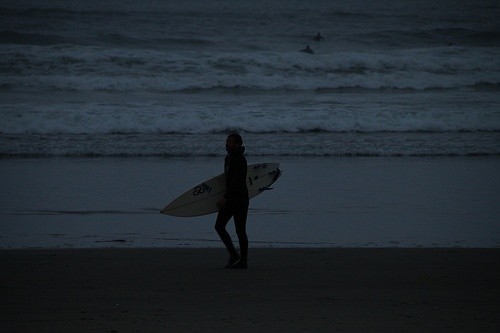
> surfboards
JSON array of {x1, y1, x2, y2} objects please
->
[{"x1": 160, "y1": 162, "x2": 282, "y2": 218}]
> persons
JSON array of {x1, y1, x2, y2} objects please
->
[{"x1": 214, "y1": 132, "x2": 251, "y2": 270}]
